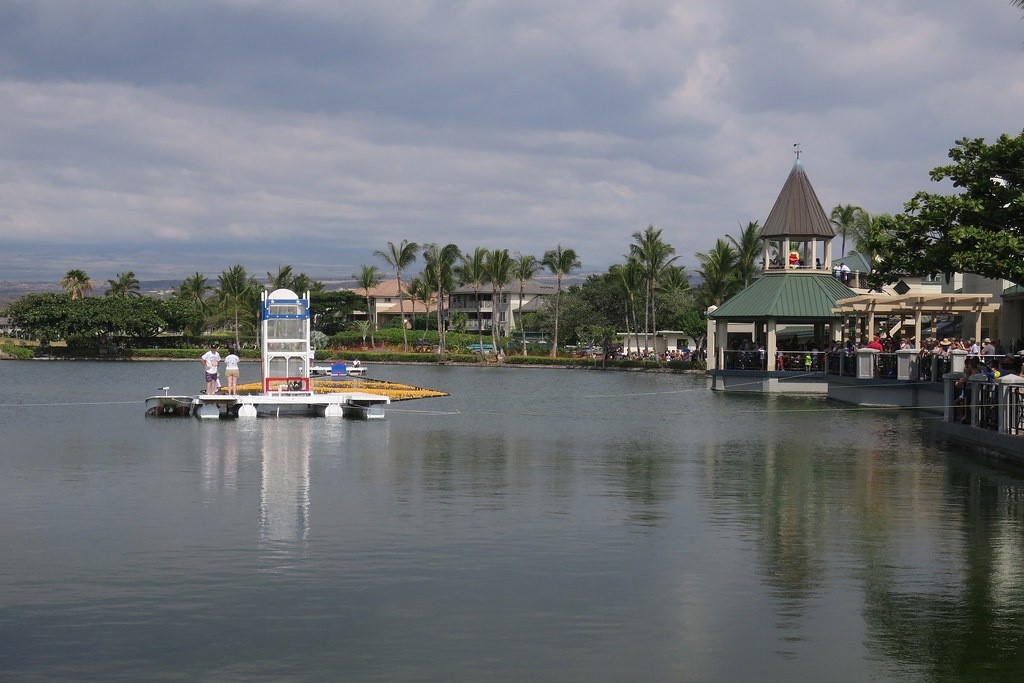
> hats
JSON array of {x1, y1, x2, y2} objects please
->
[
  {"x1": 899, "y1": 338, "x2": 906, "y2": 341},
  {"x1": 984, "y1": 338, "x2": 991, "y2": 343},
  {"x1": 970, "y1": 338, "x2": 975, "y2": 341},
  {"x1": 211, "y1": 343, "x2": 220, "y2": 349},
  {"x1": 874, "y1": 336, "x2": 881, "y2": 340},
  {"x1": 940, "y1": 338, "x2": 951, "y2": 345}
]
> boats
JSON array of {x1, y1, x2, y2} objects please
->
[{"x1": 144, "y1": 386, "x2": 196, "y2": 416}]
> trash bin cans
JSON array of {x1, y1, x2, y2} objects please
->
[{"x1": 466, "y1": 344, "x2": 494, "y2": 355}]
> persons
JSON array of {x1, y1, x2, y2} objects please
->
[
  {"x1": 224, "y1": 347, "x2": 240, "y2": 395},
  {"x1": 725, "y1": 335, "x2": 1024, "y2": 430},
  {"x1": 663, "y1": 349, "x2": 690, "y2": 361},
  {"x1": 817, "y1": 256, "x2": 851, "y2": 284},
  {"x1": 200, "y1": 342, "x2": 222, "y2": 395}
]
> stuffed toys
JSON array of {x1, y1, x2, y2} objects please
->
[{"x1": 788, "y1": 248, "x2": 799, "y2": 269}]
